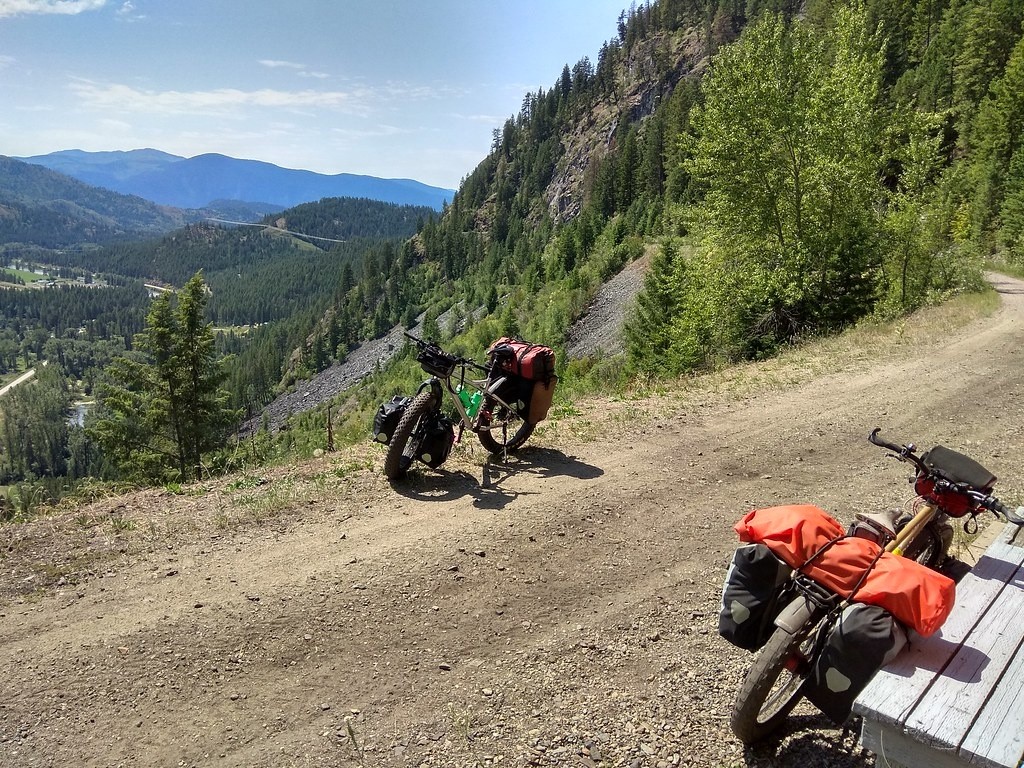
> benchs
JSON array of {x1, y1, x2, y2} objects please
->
[{"x1": 851, "y1": 506, "x2": 1024, "y2": 768}]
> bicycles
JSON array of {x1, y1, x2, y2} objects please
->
[
  {"x1": 384, "y1": 332, "x2": 536, "y2": 480},
  {"x1": 730, "y1": 427, "x2": 1024, "y2": 745}
]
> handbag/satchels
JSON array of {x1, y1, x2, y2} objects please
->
[
  {"x1": 915, "y1": 444, "x2": 998, "y2": 534},
  {"x1": 371, "y1": 395, "x2": 455, "y2": 469},
  {"x1": 487, "y1": 337, "x2": 559, "y2": 425},
  {"x1": 717, "y1": 542, "x2": 909, "y2": 726}
]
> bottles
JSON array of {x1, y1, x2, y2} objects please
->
[
  {"x1": 455, "y1": 384, "x2": 472, "y2": 408},
  {"x1": 465, "y1": 390, "x2": 482, "y2": 417}
]
